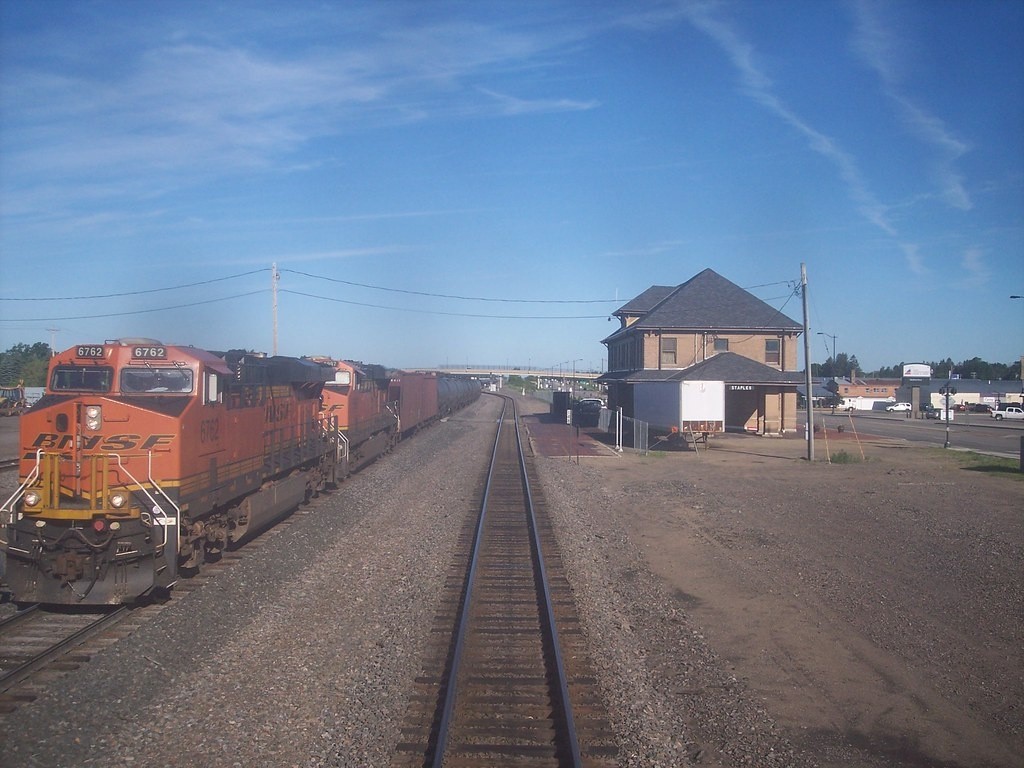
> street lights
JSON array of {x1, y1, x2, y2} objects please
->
[
  {"x1": 939, "y1": 386, "x2": 958, "y2": 449},
  {"x1": 817, "y1": 332, "x2": 838, "y2": 414},
  {"x1": 573, "y1": 358, "x2": 583, "y2": 398},
  {"x1": 551, "y1": 364, "x2": 559, "y2": 392},
  {"x1": 559, "y1": 361, "x2": 568, "y2": 391}
]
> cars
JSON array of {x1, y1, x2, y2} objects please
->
[
  {"x1": 926, "y1": 408, "x2": 943, "y2": 420},
  {"x1": 577, "y1": 398, "x2": 607, "y2": 416}
]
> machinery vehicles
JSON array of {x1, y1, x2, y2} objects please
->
[{"x1": 0, "y1": 379, "x2": 27, "y2": 417}]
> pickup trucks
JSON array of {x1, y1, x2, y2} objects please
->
[{"x1": 991, "y1": 407, "x2": 1024, "y2": 421}]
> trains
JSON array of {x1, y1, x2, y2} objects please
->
[{"x1": 0, "y1": 337, "x2": 482, "y2": 605}]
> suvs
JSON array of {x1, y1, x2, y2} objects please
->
[{"x1": 885, "y1": 402, "x2": 912, "y2": 413}]
